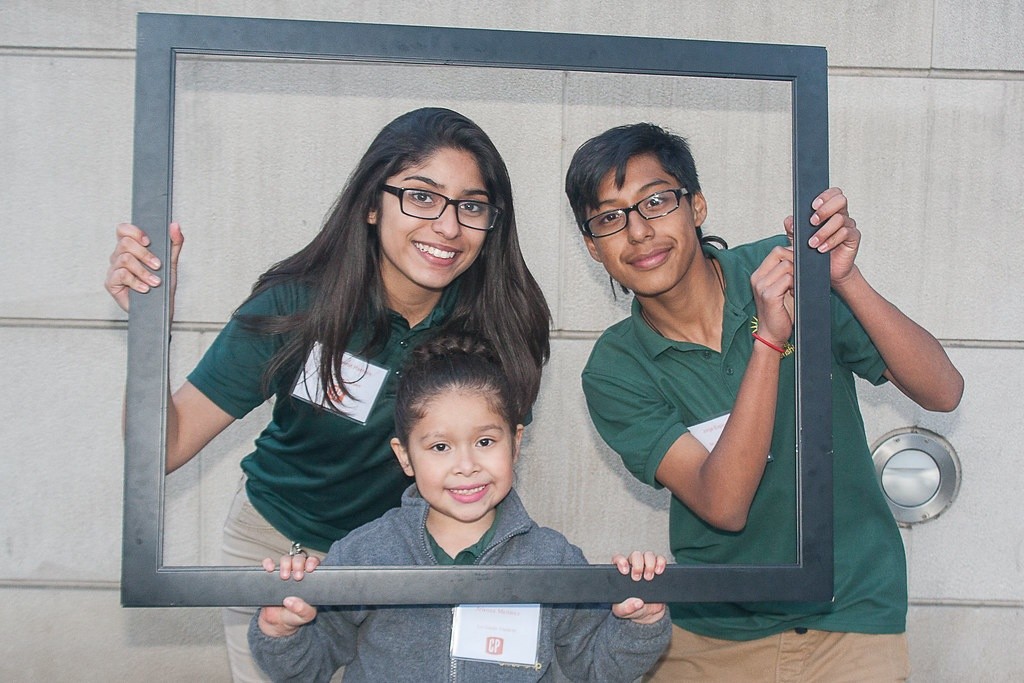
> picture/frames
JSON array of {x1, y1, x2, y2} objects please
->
[{"x1": 120, "y1": 12, "x2": 834, "y2": 608}]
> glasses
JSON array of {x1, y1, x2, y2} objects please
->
[
  {"x1": 380, "y1": 182, "x2": 504, "y2": 232},
  {"x1": 581, "y1": 188, "x2": 690, "y2": 238}
]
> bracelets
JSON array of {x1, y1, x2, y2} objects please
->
[{"x1": 754, "y1": 334, "x2": 785, "y2": 356}]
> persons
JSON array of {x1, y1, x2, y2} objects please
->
[
  {"x1": 247, "y1": 336, "x2": 674, "y2": 683},
  {"x1": 563, "y1": 124, "x2": 966, "y2": 683},
  {"x1": 104, "y1": 108, "x2": 550, "y2": 683}
]
